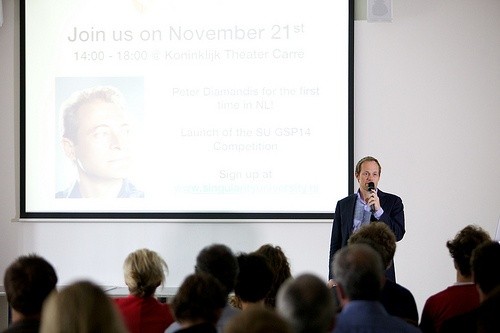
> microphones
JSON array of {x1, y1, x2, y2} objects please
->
[{"x1": 368, "y1": 182, "x2": 375, "y2": 212}]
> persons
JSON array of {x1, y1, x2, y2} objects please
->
[
  {"x1": 0, "y1": 220, "x2": 500, "y2": 333},
  {"x1": 56, "y1": 90, "x2": 147, "y2": 199},
  {"x1": 327, "y1": 156, "x2": 405, "y2": 286}
]
